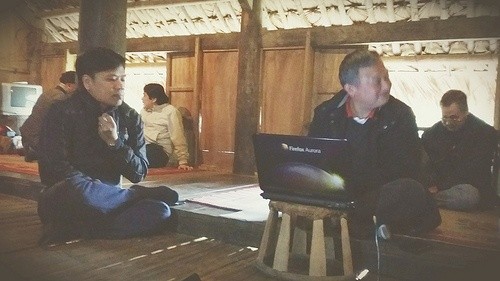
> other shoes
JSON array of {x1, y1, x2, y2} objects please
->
[
  {"x1": 25, "y1": 150, "x2": 39, "y2": 162},
  {"x1": 130, "y1": 184, "x2": 178, "y2": 206},
  {"x1": 377, "y1": 224, "x2": 391, "y2": 239},
  {"x1": 40, "y1": 205, "x2": 81, "y2": 248}
]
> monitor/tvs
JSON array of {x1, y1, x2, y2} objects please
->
[{"x1": 1, "y1": 83, "x2": 42, "y2": 116}]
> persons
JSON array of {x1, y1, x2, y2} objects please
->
[
  {"x1": 421, "y1": 90, "x2": 500, "y2": 210},
  {"x1": 36, "y1": 48, "x2": 178, "y2": 242},
  {"x1": 307, "y1": 49, "x2": 441, "y2": 238},
  {"x1": 20, "y1": 71, "x2": 77, "y2": 163},
  {"x1": 140, "y1": 83, "x2": 194, "y2": 171}
]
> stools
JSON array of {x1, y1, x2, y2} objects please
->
[{"x1": 253, "y1": 199, "x2": 356, "y2": 281}]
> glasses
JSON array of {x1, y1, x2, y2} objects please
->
[{"x1": 443, "y1": 116, "x2": 458, "y2": 121}]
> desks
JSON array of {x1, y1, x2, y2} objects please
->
[{"x1": 0, "y1": 115, "x2": 26, "y2": 155}]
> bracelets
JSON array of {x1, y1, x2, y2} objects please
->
[{"x1": 110, "y1": 144, "x2": 115, "y2": 147}]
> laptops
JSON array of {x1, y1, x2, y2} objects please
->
[{"x1": 252, "y1": 132, "x2": 362, "y2": 209}]
